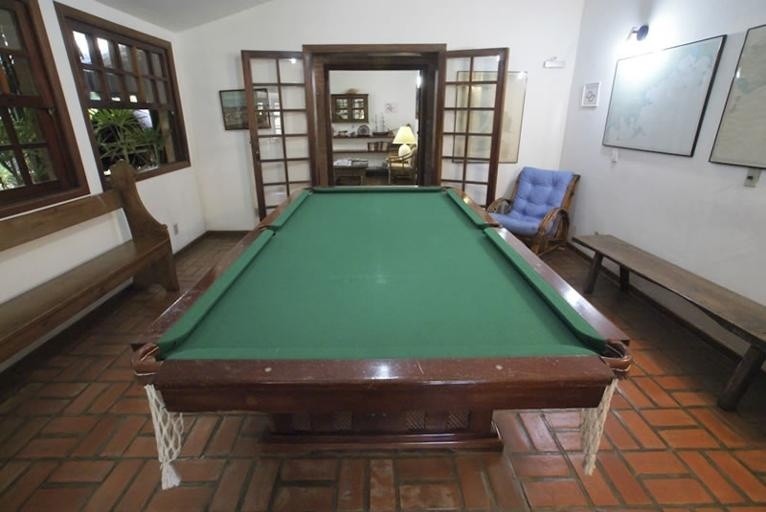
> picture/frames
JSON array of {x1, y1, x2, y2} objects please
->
[
  {"x1": 219, "y1": 88, "x2": 271, "y2": 130},
  {"x1": 581, "y1": 82, "x2": 600, "y2": 108},
  {"x1": 602, "y1": 24, "x2": 766, "y2": 170},
  {"x1": 451, "y1": 70, "x2": 528, "y2": 162}
]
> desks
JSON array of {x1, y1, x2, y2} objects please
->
[
  {"x1": 132, "y1": 185, "x2": 633, "y2": 457},
  {"x1": 333, "y1": 159, "x2": 368, "y2": 186}
]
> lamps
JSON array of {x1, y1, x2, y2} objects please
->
[{"x1": 392, "y1": 125, "x2": 416, "y2": 159}]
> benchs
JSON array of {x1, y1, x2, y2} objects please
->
[
  {"x1": 571, "y1": 234, "x2": 765, "y2": 412},
  {"x1": 0, "y1": 160, "x2": 181, "y2": 378}
]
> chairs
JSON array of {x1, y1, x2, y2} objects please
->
[
  {"x1": 485, "y1": 166, "x2": 581, "y2": 257},
  {"x1": 387, "y1": 144, "x2": 418, "y2": 184}
]
